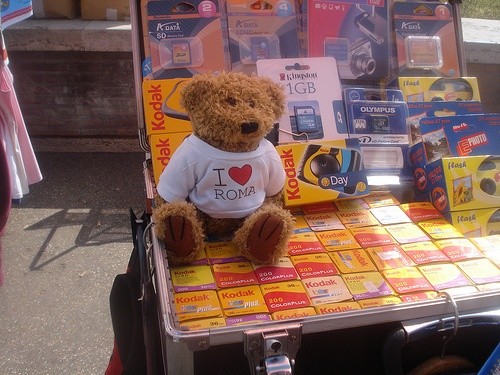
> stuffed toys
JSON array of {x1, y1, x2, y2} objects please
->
[{"x1": 154, "y1": 73, "x2": 292, "y2": 264}]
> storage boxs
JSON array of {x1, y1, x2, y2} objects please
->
[{"x1": 130, "y1": 0, "x2": 500, "y2": 375}]
